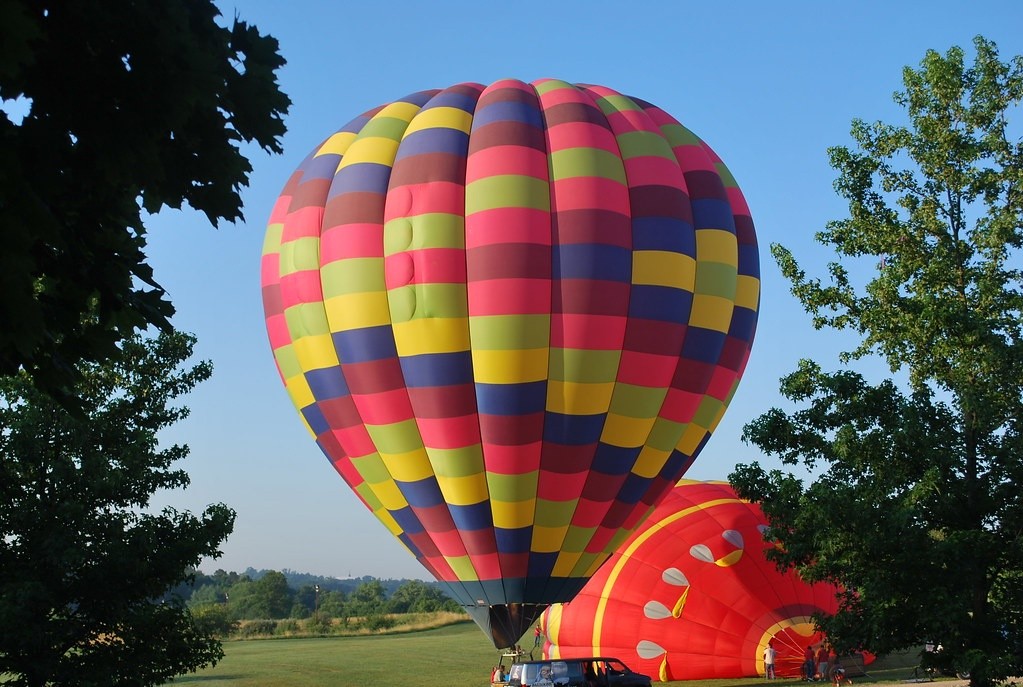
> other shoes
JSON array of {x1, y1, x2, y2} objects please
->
[
  {"x1": 808, "y1": 680, "x2": 810, "y2": 683},
  {"x1": 808, "y1": 677, "x2": 814, "y2": 681},
  {"x1": 818, "y1": 679, "x2": 821, "y2": 682},
  {"x1": 823, "y1": 679, "x2": 826, "y2": 682}
]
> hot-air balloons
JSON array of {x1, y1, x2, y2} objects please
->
[
  {"x1": 540, "y1": 477, "x2": 876, "y2": 679},
  {"x1": 260, "y1": 78, "x2": 760, "y2": 687}
]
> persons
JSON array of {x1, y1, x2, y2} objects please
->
[
  {"x1": 804, "y1": 645, "x2": 816, "y2": 682},
  {"x1": 534, "y1": 625, "x2": 541, "y2": 648},
  {"x1": 490, "y1": 665, "x2": 510, "y2": 683},
  {"x1": 763, "y1": 643, "x2": 777, "y2": 680},
  {"x1": 816, "y1": 644, "x2": 828, "y2": 681}
]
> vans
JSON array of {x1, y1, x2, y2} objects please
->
[{"x1": 508, "y1": 656, "x2": 651, "y2": 687}]
